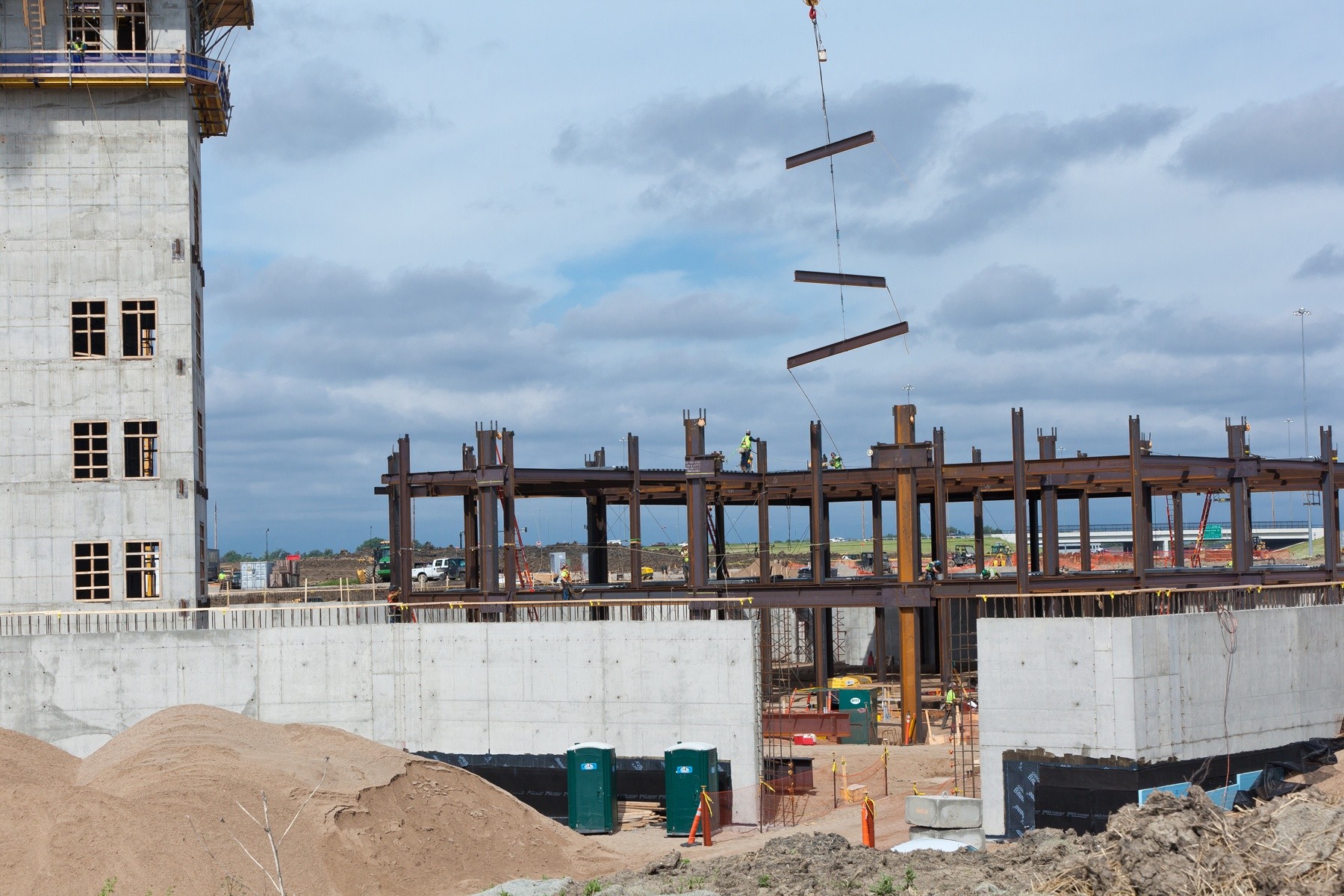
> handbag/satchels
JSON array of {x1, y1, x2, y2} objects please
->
[
  {"x1": 739, "y1": 448, "x2": 745, "y2": 453},
  {"x1": 945, "y1": 703, "x2": 951, "y2": 711},
  {"x1": 554, "y1": 575, "x2": 562, "y2": 583}
]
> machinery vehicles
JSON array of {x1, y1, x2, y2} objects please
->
[{"x1": 378, "y1": 539, "x2": 390, "y2": 582}]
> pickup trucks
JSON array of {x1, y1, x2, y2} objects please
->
[{"x1": 410, "y1": 558, "x2": 456, "y2": 583}]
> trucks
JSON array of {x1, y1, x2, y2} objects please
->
[{"x1": 436, "y1": 558, "x2": 469, "y2": 581}]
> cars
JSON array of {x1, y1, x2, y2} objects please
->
[
  {"x1": 947, "y1": 543, "x2": 976, "y2": 567},
  {"x1": 830, "y1": 537, "x2": 845, "y2": 542},
  {"x1": 641, "y1": 567, "x2": 654, "y2": 580},
  {"x1": 841, "y1": 553, "x2": 862, "y2": 569}
]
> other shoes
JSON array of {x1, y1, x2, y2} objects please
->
[
  {"x1": 222, "y1": 589, "x2": 224, "y2": 590},
  {"x1": 941, "y1": 726, "x2": 946, "y2": 730}
]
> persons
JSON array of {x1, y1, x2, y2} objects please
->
[
  {"x1": 941, "y1": 682, "x2": 956, "y2": 729},
  {"x1": 925, "y1": 561, "x2": 941, "y2": 581},
  {"x1": 680, "y1": 542, "x2": 688, "y2": 586},
  {"x1": 218, "y1": 571, "x2": 226, "y2": 590},
  {"x1": 740, "y1": 430, "x2": 759, "y2": 472},
  {"x1": 387, "y1": 585, "x2": 401, "y2": 623},
  {"x1": 979, "y1": 569, "x2": 1001, "y2": 580},
  {"x1": 830, "y1": 453, "x2": 843, "y2": 470},
  {"x1": 559, "y1": 562, "x2": 573, "y2": 606},
  {"x1": 70, "y1": 37, "x2": 87, "y2": 73}
]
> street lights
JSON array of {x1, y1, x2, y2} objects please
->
[
  {"x1": 902, "y1": 384, "x2": 915, "y2": 404},
  {"x1": 619, "y1": 437, "x2": 629, "y2": 467},
  {"x1": 1293, "y1": 307, "x2": 1313, "y2": 557}
]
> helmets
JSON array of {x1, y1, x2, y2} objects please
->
[
  {"x1": 389, "y1": 585, "x2": 398, "y2": 591},
  {"x1": 982, "y1": 570, "x2": 988, "y2": 575},
  {"x1": 561, "y1": 563, "x2": 567, "y2": 568},
  {"x1": 949, "y1": 682, "x2": 957, "y2": 687},
  {"x1": 830, "y1": 460, "x2": 835, "y2": 466},
  {"x1": 682, "y1": 543, "x2": 687, "y2": 547},
  {"x1": 936, "y1": 560, "x2": 941, "y2": 566},
  {"x1": 746, "y1": 430, "x2": 751, "y2": 434}
]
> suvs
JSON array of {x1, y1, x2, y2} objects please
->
[{"x1": 862, "y1": 551, "x2": 891, "y2": 574}]
